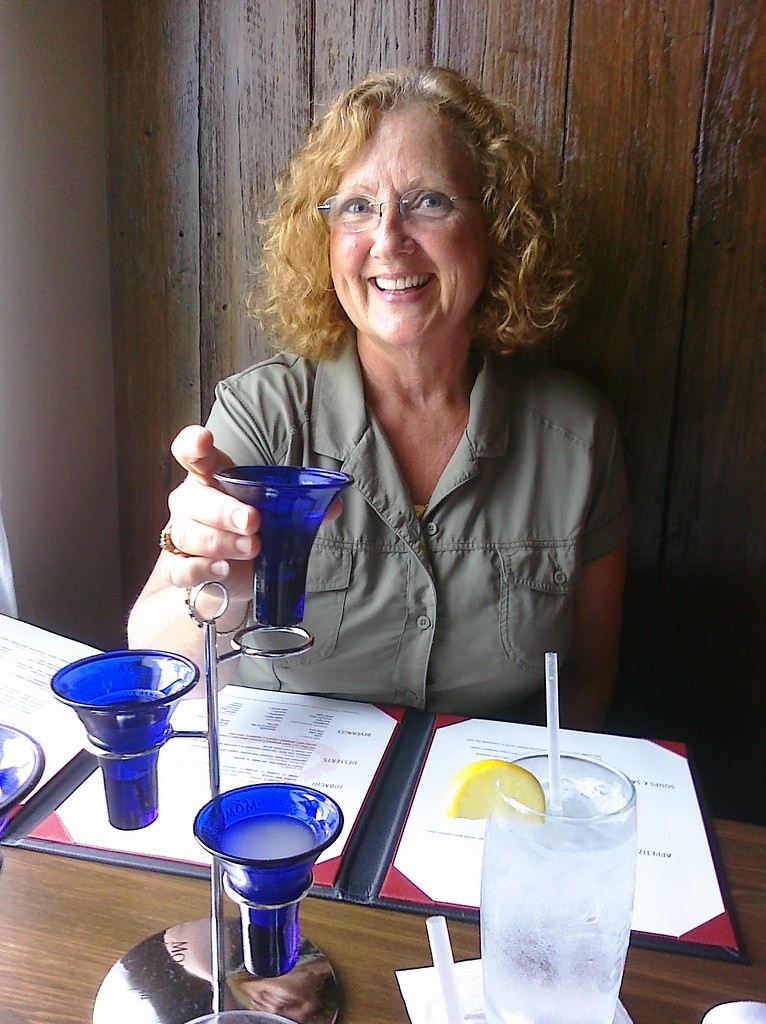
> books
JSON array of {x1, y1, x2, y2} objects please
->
[{"x1": 0, "y1": 611, "x2": 750, "y2": 966}]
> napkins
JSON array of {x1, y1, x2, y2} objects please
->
[{"x1": 395, "y1": 958, "x2": 632, "y2": 1024}]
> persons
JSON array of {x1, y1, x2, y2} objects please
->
[{"x1": 125, "y1": 66, "x2": 635, "y2": 736}]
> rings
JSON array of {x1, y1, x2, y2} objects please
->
[{"x1": 156, "y1": 525, "x2": 179, "y2": 555}]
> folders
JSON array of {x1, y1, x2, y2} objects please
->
[{"x1": 0, "y1": 680, "x2": 749, "y2": 966}]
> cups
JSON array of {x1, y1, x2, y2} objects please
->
[
  {"x1": 51, "y1": 650, "x2": 201, "y2": 832},
  {"x1": 477, "y1": 752, "x2": 640, "y2": 1024},
  {"x1": 0, "y1": 723, "x2": 46, "y2": 814},
  {"x1": 194, "y1": 780, "x2": 346, "y2": 979},
  {"x1": 211, "y1": 464, "x2": 355, "y2": 631}
]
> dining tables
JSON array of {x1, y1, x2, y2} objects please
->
[{"x1": 0, "y1": 816, "x2": 766, "y2": 1023}]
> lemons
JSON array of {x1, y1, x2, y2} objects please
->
[{"x1": 450, "y1": 759, "x2": 546, "y2": 824}]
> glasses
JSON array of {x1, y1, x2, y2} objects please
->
[{"x1": 308, "y1": 195, "x2": 487, "y2": 233}]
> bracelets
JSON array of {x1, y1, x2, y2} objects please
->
[{"x1": 181, "y1": 587, "x2": 251, "y2": 638}]
